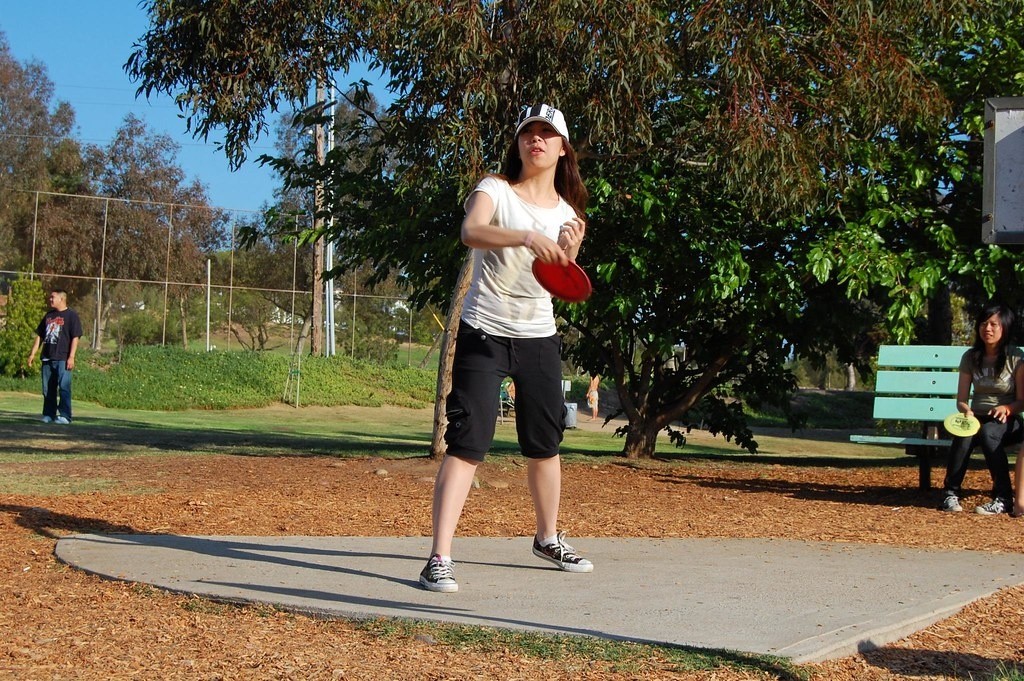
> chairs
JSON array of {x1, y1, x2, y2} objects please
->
[{"x1": 498, "y1": 381, "x2": 515, "y2": 426}]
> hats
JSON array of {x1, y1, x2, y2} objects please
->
[{"x1": 514, "y1": 103, "x2": 569, "y2": 143}]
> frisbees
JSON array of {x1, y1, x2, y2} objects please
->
[
  {"x1": 531, "y1": 255, "x2": 592, "y2": 302},
  {"x1": 944, "y1": 413, "x2": 981, "y2": 437}
]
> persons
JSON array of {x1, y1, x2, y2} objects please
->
[
  {"x1": 419, "y1": 104, "x2": 593, "y2": 591},
  {"x1": 25, "y1": 286, "x2": 84, "y2": 424},
  {"x1": 942, "y1": 304, "x2": 1024, "y2": 515},
  {"x1": 586, "y1": 374, "x2": 600, "y2": 422}
]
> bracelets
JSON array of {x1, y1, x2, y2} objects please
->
[{"x1": 525, "y1": 231, "x2": 537, "y2": 248}]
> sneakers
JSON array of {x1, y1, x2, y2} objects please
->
[
  {"x1": 532, "y1": 531, "x2": 594, "y2": 572},
  {"x1": 54, "y1": 416, "x2": 70, "y2": 424},
  {"x1": 975, "y1": 497, "x2": 1014, "y2": 515},
  {"x1": 420, "y1": 554, "x2": 459, "y2": 593},
  {"x1": 942, "y1": 491, "x2": 962, "y2": 511},
  {"x1": 41, "y1": 415, "x2": 52, "y2": 423}
]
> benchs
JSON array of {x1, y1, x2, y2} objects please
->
[{"x1": 849, "y1": 345, "x2": 1024, "y2": 490}]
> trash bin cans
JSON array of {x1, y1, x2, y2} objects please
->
[{"x1": 564, "y1": 402, "x2": 577, "y2": 428}]
